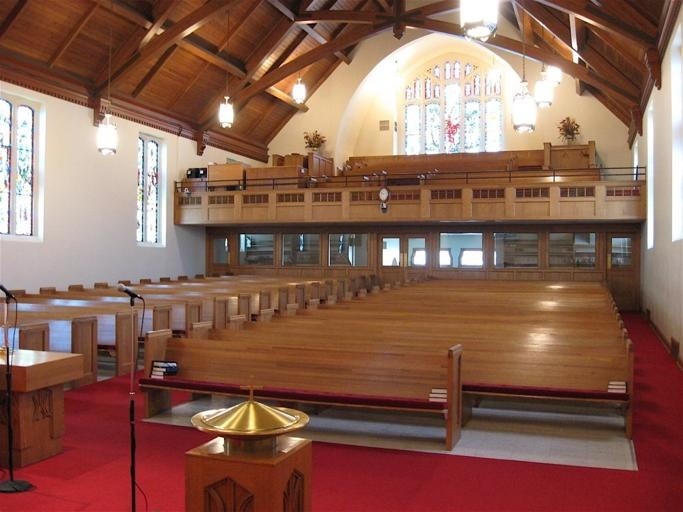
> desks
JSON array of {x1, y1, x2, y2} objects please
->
[{"x1": 0, "y1": 351, "x2": 88, "y2": 474}]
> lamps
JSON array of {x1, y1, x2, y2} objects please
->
[
  {"x1": 218, "y1": 9, "x2": 234, "y2": 128},
  {"x1": 534, "y1": 24, "x2": 553, "y2": 108},
  {"x1": 95, "y1": 6, "x2": 117, "y2": 156},
  {"x1": 460, "y1": 0, "x2": 499, "y2": 43},
  {"x1": 292, "y1": 70, "x2": 306, "y2": 104},
  {"x1": 511, "y1": 11, "x2": 537, "y2": 132}
]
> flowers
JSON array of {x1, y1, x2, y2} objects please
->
[
  {"x1": 303, "y1": 130, "x2": 327, "y2": 148},
  {"x1": 556, "y1": 117, "x2": 581, "y2": 144}
]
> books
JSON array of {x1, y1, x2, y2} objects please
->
[{"x1": 150, "y1": 360, "x2": 179, "y2": 381}]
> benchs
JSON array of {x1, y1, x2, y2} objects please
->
[{"x1": 0, "y1": 270, "x2": 634, "y2": 451}]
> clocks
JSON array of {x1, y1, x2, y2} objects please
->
[{"x1": 380, "y1": 188, "x2": 389, "y2": 201}]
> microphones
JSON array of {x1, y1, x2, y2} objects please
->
[
  {"x1": 0, "y1": 284, "x2": 18, "y2": 302},
  {"x1": 117, "y1": 283, "x2": 144, "y2": 300}
]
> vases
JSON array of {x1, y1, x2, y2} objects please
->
[
  {"x1": 567, "y1": 135, "x2": 576, "y2": 145},
  {"x1": 310, "y1": 148, "x2": 319, "y2": 155}
]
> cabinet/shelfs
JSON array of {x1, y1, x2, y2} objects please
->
[{"x1": 181, "y1": 154, "x2": 334, "y2": 191}]
